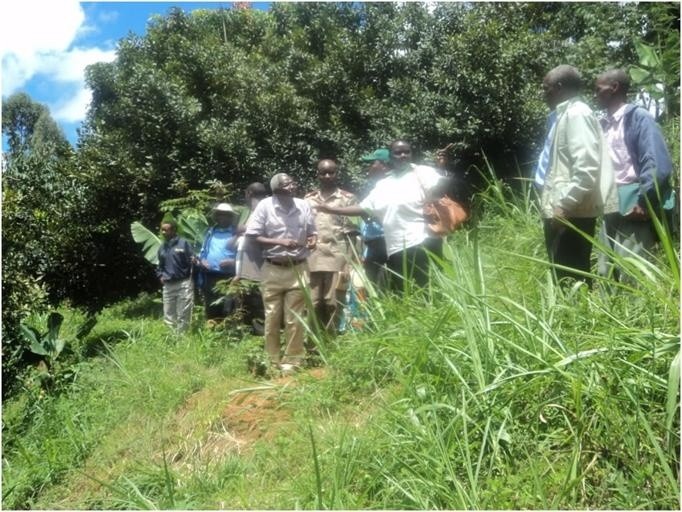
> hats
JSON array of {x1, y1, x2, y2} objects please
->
[
  {"x1": 213, "y1": 203, "x2": 239, "y2": 216},
  {"x1": 362, "y1": 149, "x2": 390, "y2": 162}
]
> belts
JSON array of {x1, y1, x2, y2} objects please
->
[{"x1": 266, "y1": 259, "x2": 304, "y2": 266}]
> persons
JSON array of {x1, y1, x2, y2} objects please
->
[
  {"x1": 199, "y1": 182, "x2": 268, "y2": 321},
  {"x1": 593, "y1": 69, "x2": 673, "y2": 294},
  {"x1": 531, "y1": 64, "x2": 605, "y2": 299},
  {"x1": 305, "y1": 159, "x2": 360, "y2": 335},
  {"x1": 154, "y1": 220, "x2": 200, "y2": 333},
  {"x1": 244, "y1": 173, "x2": 317, "y2": 373},
  {"x1": 311, "y1": 141, "x2": 451, "y2": 300}
]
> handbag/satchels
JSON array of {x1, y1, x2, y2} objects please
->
[
  {"x1": 424, "y1": 194, "x2": 467, "y2": 235},
  {"x1": 194, "y1": 265, "x2": 206, "y2": 289},
  {"x1": 617, "y1": 183, "x2": 675, "y2": 217},
  {"x1": 364, "y1": 237, "x2": 387, "y2": 263}
]
